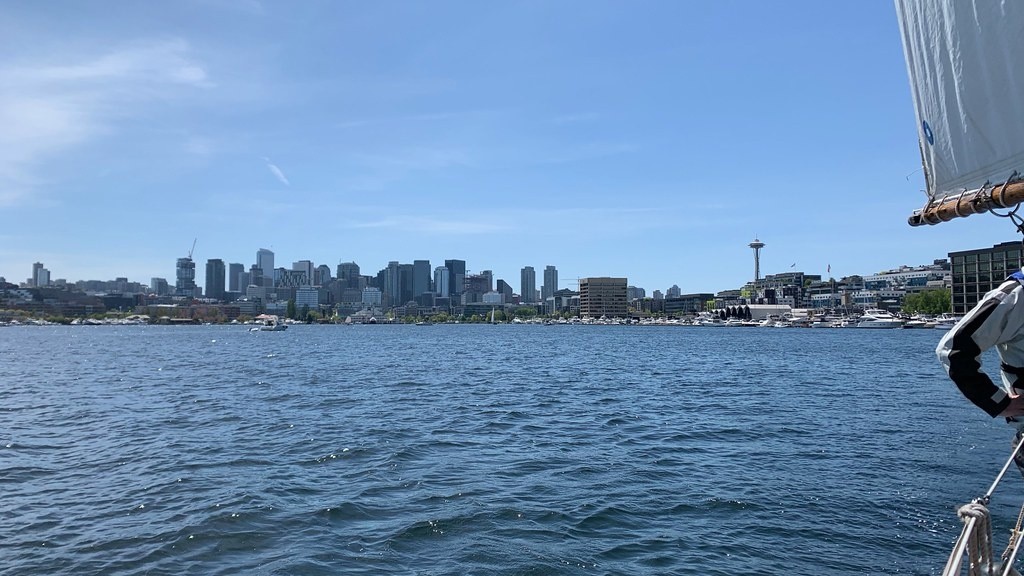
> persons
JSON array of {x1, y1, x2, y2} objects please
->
[{"x1": 936, "y1": 264, "x2": 1024, "y2": 477}]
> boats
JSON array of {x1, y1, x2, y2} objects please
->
[
  {"x1": 259, "y1": 318, "x2": 288, "y2": 331},
  {"x1": 0, "y1": 314, "x2": 149, "y2": 327},
  {"x1": 508, "y1": 308, "x2": 960, "y2": 330},
  {"x1": 415, "y1": 322, "x2": 432, "y2": 326}
]
